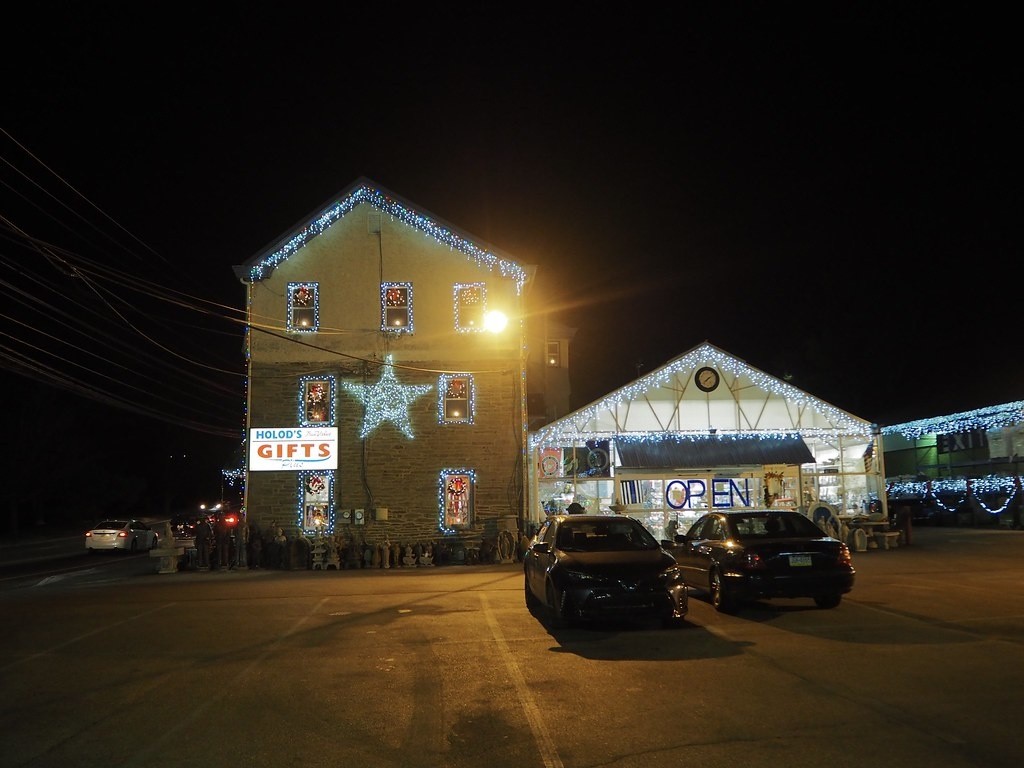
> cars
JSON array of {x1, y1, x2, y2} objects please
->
[
  {"x1": 169, "y1": 509, "x2": 242, "y2": 540},
  {"x1": 84, "y1": 518, "x2": 158, "y2": 554},
  {"x1": 522, "y1": 513, "x2": 689, "y2": 629},
  {"x1": 659, "y1": 508, "x2": 857, "y2": 612}
]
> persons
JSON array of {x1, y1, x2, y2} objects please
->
[{"x1": 193, "y1": 513, "x2": 231, "y2": 569}]
